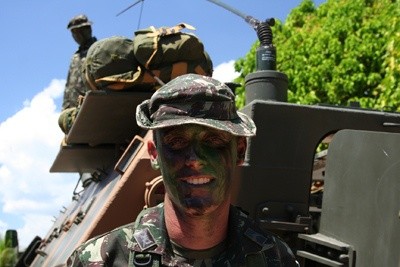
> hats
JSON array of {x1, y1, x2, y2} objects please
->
[
  {"x1": 66, "y1": 13, "x2": 93, "y2": 31},
  {"x1": 135, "y1": 73, "x2": 257, "y2": 138}
]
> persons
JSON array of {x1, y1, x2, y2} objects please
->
[
  {"x1": 62, "y1": 15, "x2": 98, "y2": 110},
  {"x1": 64, "y1": 73, "x2": 301, "y2": 267}
]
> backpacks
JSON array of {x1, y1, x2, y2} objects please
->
[{"x1": 85, "y1": 22, "x2": 212, "y2": 83}]
